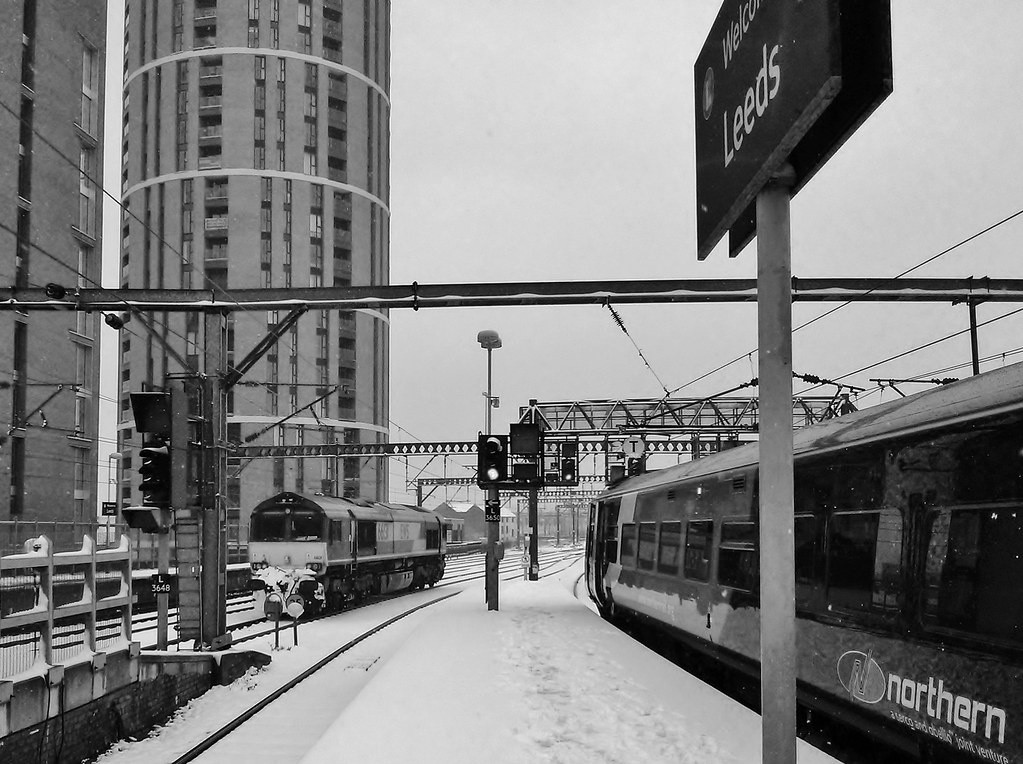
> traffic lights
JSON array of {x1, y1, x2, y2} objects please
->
[
  {"x1": 479, "y1": 432, "x2": 509, "y2": 483},
  {"x1": 511, "y1": 421, "x2": 541, "y2": 456},
  {"x1": 560, "y1": 457, "x2": 576, "y2": 483},
  {"x1": 562, "y1": 442, "x2": 576, "y2": 458},
  {"x1": 138, "y1": 446, "x2": 172, "y2": 506},
  {"x1": 128, "y1": 388, "x2": 172, "y2": 442}
]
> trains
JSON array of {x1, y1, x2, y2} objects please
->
[
  {"x1": 579, "y1": 353, "x2": 1023, "y2": 764},
  {"x1": 248, "y1": 489, "x2": 446, "y2": 613}
]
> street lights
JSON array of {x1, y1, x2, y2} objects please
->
[
  {"x1": 477, "y1": 328, "x2": 503, "y2": 611},
  {"x1": 108, "y1": 453, "x2": 123, "y2": 546}
]
